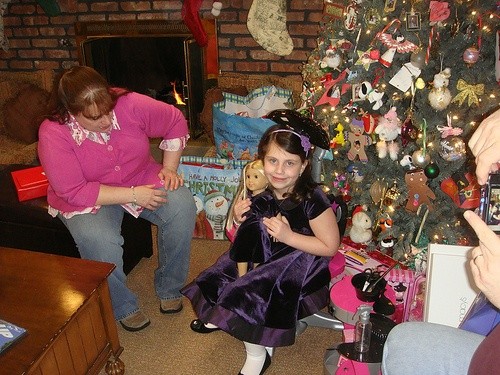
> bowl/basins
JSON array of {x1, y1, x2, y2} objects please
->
[{"x1": 351, "y1": 272, "x2": 387, "y2": 303}]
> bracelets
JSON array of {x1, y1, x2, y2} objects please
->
[{"x1": 131, "y1": 186, "x2": 137, "y2": 204}]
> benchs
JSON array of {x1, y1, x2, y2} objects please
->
[{"x1": 1, "y1": 164, "x2": 154, "y2": 276}]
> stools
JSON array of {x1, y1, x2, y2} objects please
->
[{"x1": 330, "y1": 276, "x2": 396, "y2": 375}]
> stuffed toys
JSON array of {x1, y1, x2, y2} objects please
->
[{"x1": 297, "y1": 5, "x2": 451, "y2": 243}]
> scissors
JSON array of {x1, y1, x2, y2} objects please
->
[{"x1": 362, "y1": 268, "x2": 379, "y2": 292}]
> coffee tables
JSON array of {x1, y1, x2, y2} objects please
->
[{"x1": 0, "y1": 248, "x2": 125, "y2": 375}]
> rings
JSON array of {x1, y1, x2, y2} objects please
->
[{"x1": 473, "y1": 254, "x2": 483, "y2": 265}]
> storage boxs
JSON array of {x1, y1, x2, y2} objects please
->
[{"x1": 11, "y1": 167, "x2": 50, "y2": 201}]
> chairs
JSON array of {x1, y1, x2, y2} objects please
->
[{"x1": 266, "y1": 109, "x2": 344, "y2": 332}]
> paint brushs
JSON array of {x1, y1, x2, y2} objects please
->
[{"x1": 367, "y1": 261, "x2": 399, "y2": 292}]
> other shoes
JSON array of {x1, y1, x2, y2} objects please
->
[
  {"x1": 190, "y1": 318, "x2": 224, "y2": 334},
  {"x1": 237, "y1": 347, "x2": 271, "y2": 375},
  {"x1": 160, "y1": 297, "x2": 183, "y2": 314},
  {"x1": 119, "y1": 309, "x2": 151, "y2": 332}
]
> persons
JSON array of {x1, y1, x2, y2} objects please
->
[
  {"x1": 180, "y1": 124, "x2": 339, "y2": 375},
  {"x1": 381, "y1": 108, "x2": 500, "y2": 375},
  {"x1": 225, "y1": 160, "x2": 280, "y2": 278},
  {"x1": 37, "y1": 66, "x2": 196, "y2": 331}
]
